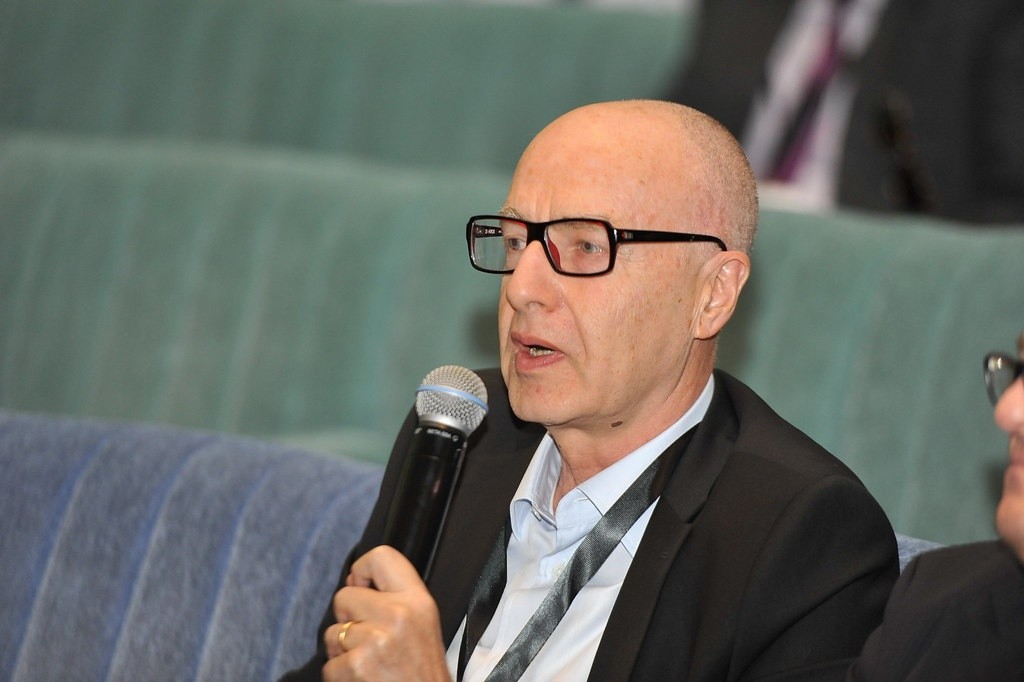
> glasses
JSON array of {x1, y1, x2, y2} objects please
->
[
  {"x1": 466, "y1": 215, "x2": 727, "y2": 276},
  {"x1": 983, "y1": 353, "x2": 1024, "y2": 404}
]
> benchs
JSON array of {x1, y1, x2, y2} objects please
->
[{"x1": 0, "y1": 0, "x2": 1024, "y2": 682}]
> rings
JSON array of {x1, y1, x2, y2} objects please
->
[{"x1": 338, "y1": 620, "x2": 358, "y2": 653}]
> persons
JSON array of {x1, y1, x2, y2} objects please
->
[
  {"x1": 843, "y1": 328, "x2": 1024, "y2": 682},
  {"x1": 667, "y1": 0, "x2": 1024, "y2": 235},
  {"x1": 275, "y1": 98, "x2": 903, "y2": 682}
]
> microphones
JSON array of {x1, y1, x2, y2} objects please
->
[{"x1": 367, "y1": 366, "x2": 487, "y2": 593}]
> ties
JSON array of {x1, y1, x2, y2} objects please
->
[{"x1": 768, "y1": 0, "x2": 856, "y2": 182}]
